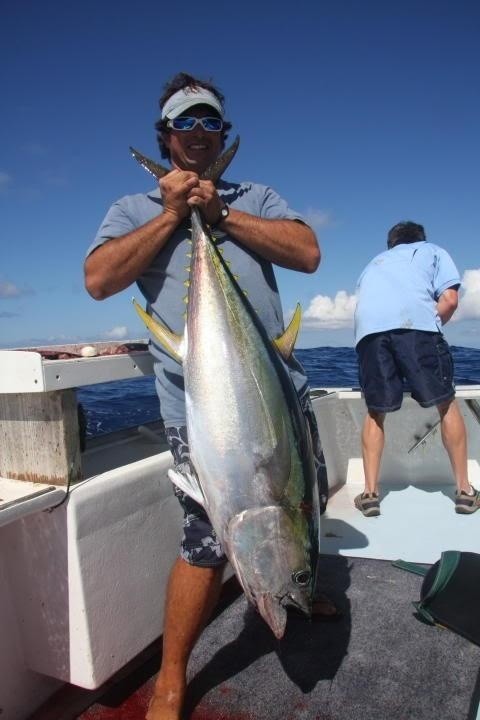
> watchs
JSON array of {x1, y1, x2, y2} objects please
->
[{"x1": 209, "y1": 203, "x2": 229, "y2": 227}]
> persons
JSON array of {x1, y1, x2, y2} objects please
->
[
  {"x1": 84, "y1": 73, "x2": 326, "y2": 720},
  {"x1": 349, "y1": 219, "x2": 480, "y2": 515}
]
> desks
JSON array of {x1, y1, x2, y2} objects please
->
[{"x1": 0, "y1": 338, "x2": 161, "y2": 483}]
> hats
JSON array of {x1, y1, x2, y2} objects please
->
[{"x1": 160, "y1": 85, "x2": 225, "y2": 118}]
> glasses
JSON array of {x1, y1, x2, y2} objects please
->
[{"x1": 167, "y1": 116, "x2": 226, "y2": 133}]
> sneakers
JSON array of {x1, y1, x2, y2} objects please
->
[
  {"x1": 354, "y1": 492, "x2": 380, "y2": 518},
  {"x1": 455, "y1": 484, "x2": 477, "y2": 514}
]
